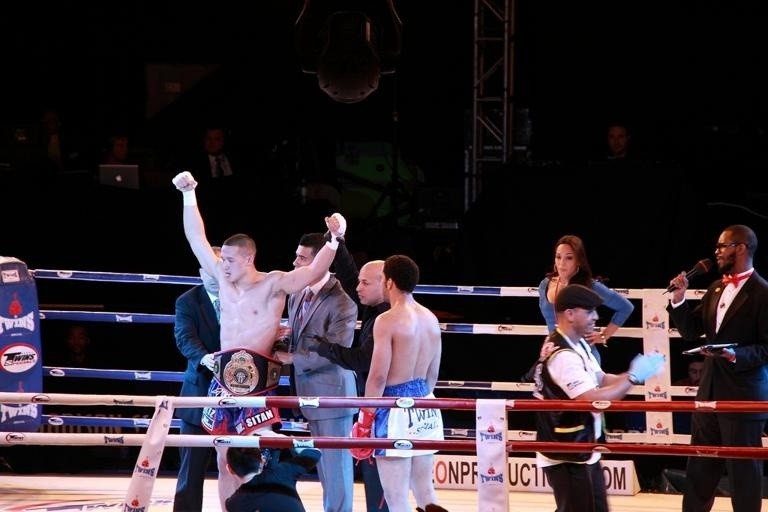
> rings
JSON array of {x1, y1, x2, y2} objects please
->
[{"x1": 599, "y1": 330, "x2": 610, "y2": 349}]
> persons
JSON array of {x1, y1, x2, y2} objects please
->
[
  {"x1": 588, "y1": 120, "x2": 670, "y2": 288},
  {"x1": 171, "y1": 170, "x2": 349, "y2": 512},
  {"x1": 223, "y1": 431, "x2": 323, "y2": 512},
  {"x1": 534, "y1": 284, "x2": 646, "y2": 512},
  {"x1": 537, "y1": 235, "x2": 634, "y2": 368},
  {"x1": 171, "y1": 265, "x2": 293, "y2": 512},
  {"x1": 32, "y1": 108, "x2": 83, "y2": 183},
  {"x1": 42, "y1": 326, "x2": 128, "y2": 415},
  {"x1": 318, "y1": 260, "x2": 390, "y2": 512},
  {"x1": 273, "y1": 233, "x2": 359, "y2": 512},
  {"x1": 351, "y1": 254, "x2": 449, "y2": 512},
  {"x1": 94, "y1": 132, "x2": 145, "y2": 191},
  {"x1": 196, "y1": 129, "x2": 254, "y2": 246},
  {"x1": 666, "y1": 224, "x2": 768, "y2": 512},
  {"x1": 672, "y1": 356, "x2": 705, "y2": 471}
]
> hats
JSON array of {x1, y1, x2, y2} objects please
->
[{"x1": 555, "y1": 286, "x2": 604, "y2": 311}]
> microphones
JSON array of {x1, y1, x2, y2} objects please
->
[{"x1": 664, "y1": 257, "x2": 716, "y2": 298}]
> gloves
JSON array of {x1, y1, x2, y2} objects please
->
[
  {"x1": 629, "y1": 353, "x2": 665, "y2": 383},
  {"x1": 302, "y1": 334, "x2": 325, "y2": 352},
  {"x1": 350, "y1": 407, "x2": 376, "y2": 460}
]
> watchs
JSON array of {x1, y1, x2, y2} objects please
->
[{"x1": 627, "y1": 372, "x2": 641, "y2": 386}]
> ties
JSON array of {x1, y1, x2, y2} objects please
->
[
  {"x1": 295, "y1": 290, "x2": 313, "y2": 339},
  {"x1": 212, "y1": 299, "x2": 221, "y2": 323}
]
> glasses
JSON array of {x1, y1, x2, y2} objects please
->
[{"x1": 716, "y1": 242, "x2": 739, "y2": 248}]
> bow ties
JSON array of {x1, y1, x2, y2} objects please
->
[{"x1": 722, "y1": 273, "x2": 739, "y2": 288}]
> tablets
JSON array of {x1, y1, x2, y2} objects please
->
[{"x1": 683, "y1": 343, "x2": 739, "y2": 357}]
